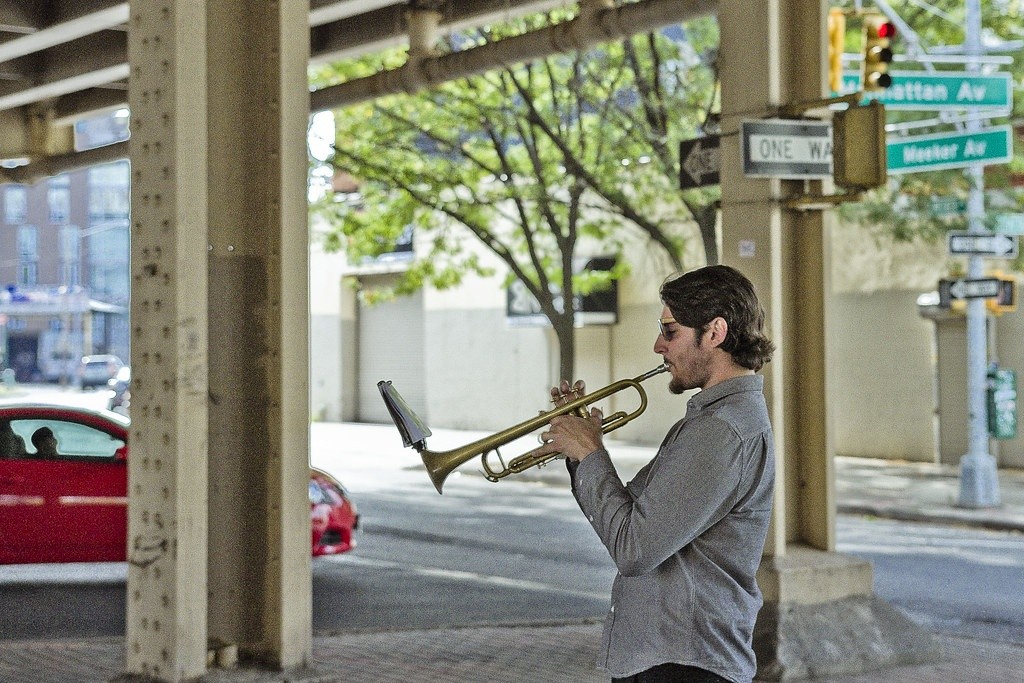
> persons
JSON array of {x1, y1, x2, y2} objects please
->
[
  {"x1": 531, "y1": 264, "x2": 779, "y2": 683},
  {"x1": 30, "y1": 426, "x2": 60, "y2": 456}
]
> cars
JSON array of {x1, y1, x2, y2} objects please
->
[
  {"x1": 78, "y1": 354, "x2": 125, "y2": 392},
  {"x1": 106, "y1": 368, "x2": 133, "y2": 413},
  {"x1": 0, "y1": 403, "x2": 362, "y2": 564}
]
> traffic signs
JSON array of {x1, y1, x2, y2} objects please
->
[{"x1": 944, "y1": 228, "x2": 1022, "y2": 263}]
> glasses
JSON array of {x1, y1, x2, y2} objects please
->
[{"x1": 657, "y1": 317, "x2": 678, "y2": 342}]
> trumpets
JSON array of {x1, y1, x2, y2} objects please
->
[{"x1": 417, "y1": 360, "x2": 673, "y2": 498}]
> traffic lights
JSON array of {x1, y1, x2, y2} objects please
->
[{"x1": 861, "y1": 15, "x2": 897, "y2": 95}]
